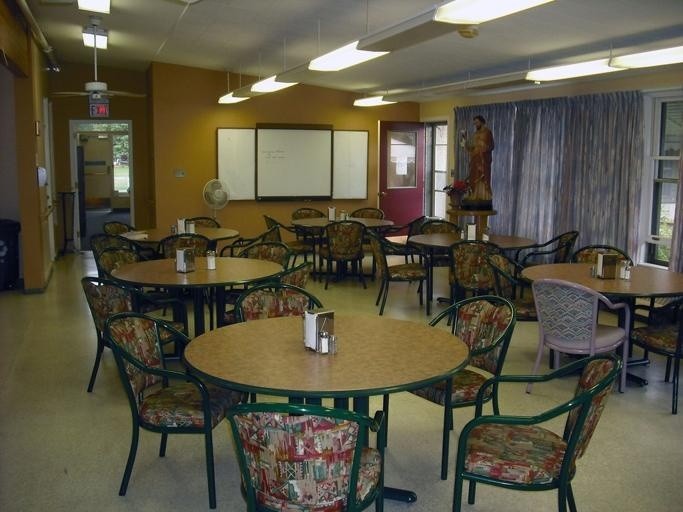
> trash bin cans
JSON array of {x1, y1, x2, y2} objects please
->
[{"x1": 0, "y1": 217, "x2": 21, "y2": 291}]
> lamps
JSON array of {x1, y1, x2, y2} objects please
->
[
  {"x1": 214, "y1": 65, "x2": 249, "y2": 104},
  {"x1": 75, "y1": 0, "x2": 113, "y2": 13},
  {"x1": 523, "y1": 56, "x2": 626, "y2": 83},
  {"x1": 606, "y1": 44, "x2": 683, "y2": 69},
  {"x1": 79, "y1": 32, "x2": 110, "y2": 51},
  {"x1": 426, "y1": 0, "x2": 551, "y2": 25},
  {"x1": 347, "y1": 88, "x2": 399, "y2": 107},
  {"x1": 305, "y1": 0, "x2": 390, "y2": 71},
  {"x1": 245, "y1": 40, "x2": 297, "y2": 95}
]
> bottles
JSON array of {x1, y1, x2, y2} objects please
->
[
  {"x1": 329, "y1": 335, "x2": 337, "y2": 354},
  {"x1": 319, "y1": 332, "x2": 329, "y2": 354},
  {"x1": 340, "y1": 209, "x2": 346, "y2": 220},
  {"x1": 185, "y1": 221, "x2": 189, "y2": 232},
  {"x1": 206, "y1": 250, "x2": 215, "y2": 270},
  {"x1": 620, "y1": 260, "x2": 626, "y2": 279},
  {"x1": 625, "y1": 267, "x2": 632, "y2": 281},
  {"x1": 461, "y1": 229, "x2": 464, "y2": 240},
  {"x1": 190, "y1": 221, "x2": 195, "y2": 232}
]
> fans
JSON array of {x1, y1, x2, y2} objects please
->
[
  {"x1": 202, "y1": 179, "x2": 230, "y2": 216},
  {"x1": 51, "y1": 15, "x2": 150, "y2": 102}
]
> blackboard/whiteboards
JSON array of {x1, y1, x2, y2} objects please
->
[{"x1": 215, "y1": 127, "x2": 368, "y2": 201}]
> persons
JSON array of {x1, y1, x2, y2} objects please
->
[{"x1": 461, "y1": 113, "x2": 495, "y2": 200}]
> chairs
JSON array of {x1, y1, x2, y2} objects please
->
[
  {"x1": 515, "y1": 230, "x2": 580, "y2": 301},
  {"x1": 93, "y1": 247, "x2": 146, "y2": 277},
  {"x1": 236, "y1": 240, "x2": 290, "y2": 266},
  {"x1": 291, "y1": 207, "x2": 326, "y2": 220},
  {"x1": 263, "y1": 216, "x2": 313, "y2": 270},
  {"x1": 528, "y1": 279, "x2": 631, "y2": 393},
  {"x1": 631, "y1": 303, "x2": 683, "y2": 413},
  {"x1": 157, "y1": 233, "x2": 210, "y2": 257},
  {"x1": 255, "y1": 261, "x2": 314, "y2": 286},
  {"x1": 89, "y1": 233, "x2": 154, "y2": 254},
  {"x1": 572, "y1": 243, "x2": 633, "y2": 267},
  {"x1": 81, "y1": 276, "x2": 187, "y2": 394},
  {"x1": 181, "y1": 216, "x2": 220, "y2": 228},
  {"x1": 349, "y1": 207, "x2": 384, "y2": 219},
  {"x1": 420, "y1": 219, "x2": 459, "y2": 235},
  {"x1": 369, "y1": 236, "x2": 430, "y2": 315},
  {"x1": 234, "y1": 284, "x2": 323, "y2": 321},
  {"x1": 386, "y1": 216, "x2": 425, "y2": 264},
  {"x1": 104, "y1": 220, "x2": 136, "y2": 235},
  {"x1": 450, "y1": 353, "x2": 623, "y2": 512},
  {"x1": 318, "y1": 219, "x2": 369, "y2": 290},
  {"x1": 446, "y1": 240, "x2": 503, "y2": 322},
  {"x1": 102, "y1": 309, "x2": 246, "y2": 509},
  {"x1": 225, "y1": 403, "x2": 385, "y2": 511},
  {"x1": 398, "y1": 296, "x2": 519, "y2": 416}
]
